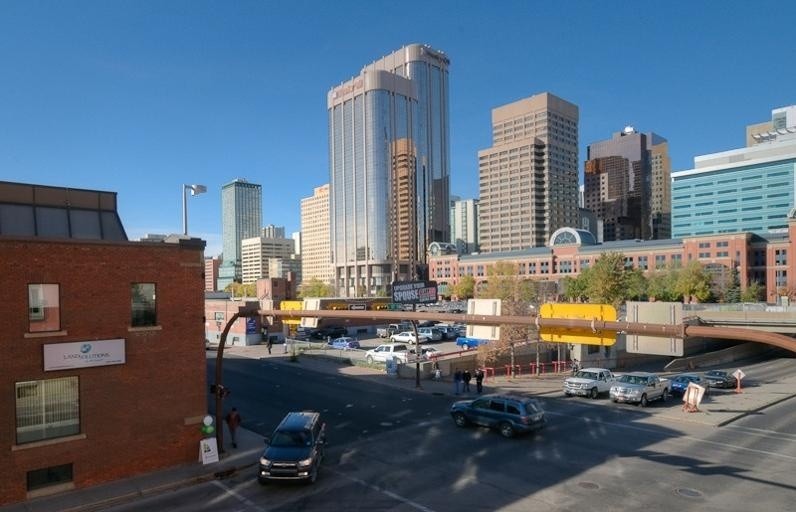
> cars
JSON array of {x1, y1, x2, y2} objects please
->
[
  {"x1": 610, "y1": 370, "x2": 737, "y2": 406},
  {"x1": 289, "y1": 321, "x2": 487, "y2": 365}
]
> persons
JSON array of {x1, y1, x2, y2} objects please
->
[
  {"x1": 462, "y1": 369, "x2": 471, "y2": 393},
  {"x1": 474, "y1": 369, "x2": 484, "y2": 395},
  {"x1": 225, "y1": 407, "x2": 243, "y2": 449},
  {"x1": 454, "y1": 367, "x2": 462, "y2": 395},
  {"x1": 573, "y1": 359, "x2": 577, "y2": 375},
  {"x1": 430, "y1": 358, "x2": 438, "y2": 381},
  {"x1": 266, "y1": 339, "x2": 272, "y2": 354}
]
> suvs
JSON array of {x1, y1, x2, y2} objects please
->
[
  {"x1": 563, "y1": 367, "x2": 621, "y2": 399},
  {"x1": 257, "y1": 410, "x2": 327, "y2": 485},
  {"x1": 450, "y1": 393, "x2": 547, "y2": 437}
]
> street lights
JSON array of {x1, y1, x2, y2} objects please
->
[{"x1": 180, "y1": 181, "x2": 206, "y2": 236}]
